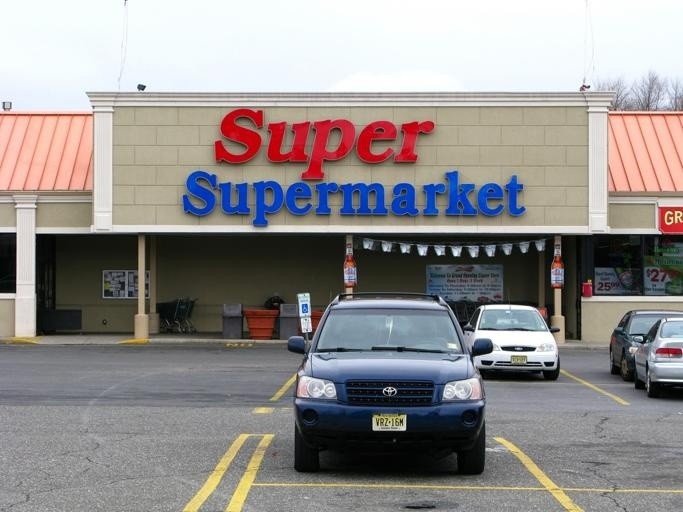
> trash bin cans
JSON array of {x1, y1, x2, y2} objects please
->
[
  {"x1": 222, "y1": 303, "x2": 244, "y2": 339},
  {"x1": 279, "y1": 304, "x2": 299, "y2": 340}
]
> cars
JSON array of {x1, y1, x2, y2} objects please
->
[
  {"x1": 609, "y1": 309, "x2": 683, "y2": 381},
  {"x1": 632, "y1": 316, "x2": 682, "y2": 397},
  {"x1": 463, "y1": 302, "x2": 560, "y2": 381}
]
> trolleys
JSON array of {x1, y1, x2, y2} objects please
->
[
  {"x1": 165, "y1": 298, "x2": 198, "y2": 335},
  {"x1": 155, "y1": 296, "x2": 185, "y2": 336}
]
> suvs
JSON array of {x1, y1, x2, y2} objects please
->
[{"x1": 286, "y1": 290, "x2": 493, "y2": 475}]
